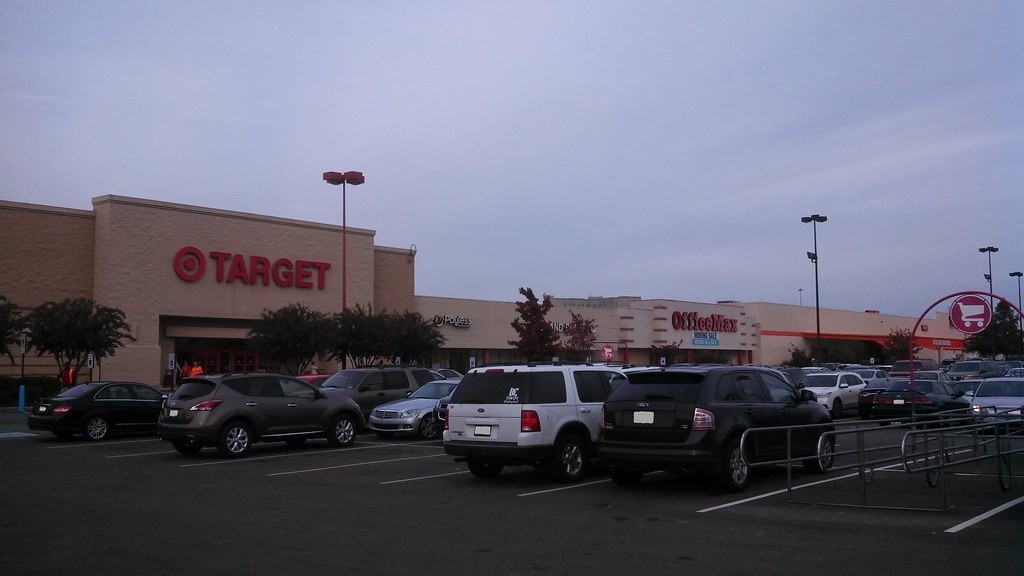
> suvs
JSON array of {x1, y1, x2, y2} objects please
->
[
  {"x1": 157, "y1": 371, "x2": 366, "y2": 459},
  {"x1": 441, "y1": 359, "x2": 628, "y2": 482},
  {"x1": 321, "y1": 363, "x2": 441, "y2": 431},
  {"x1": 594, "y1": 363, "x2": 838, "y2": 497}
]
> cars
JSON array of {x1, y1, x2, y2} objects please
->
[
  {"x1": 28, "y1": 379, "x2": 167, "y2": 442},
  {"x1": 597, "y1": 351, "x2": 1024, "y2": 436},
  {"x1": 428, "y1": 366, "x2": 464, "y2": 380},
  {"x1": 285, "y1": 374, "x2": 332, "y2": 393},
  {"x1": 367, "y1": 378, "x2": 462, "y2": 441}
]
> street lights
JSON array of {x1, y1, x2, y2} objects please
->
[
  {"x1": 801, "y1": 213, "x2": 828, "y2": 367},
  {"x1": 1009, "y1": 271, "x2": 1024, "y2": 355},
  {"x1": 978, "y1": 246, "x2": 999, "y2": 361},
  {"x1": 321, "y1": 169, "x2": 367, "y2": 370}
]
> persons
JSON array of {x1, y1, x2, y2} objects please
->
[
  {"x1": 310, "y1": 361, "x2": 317, "y2": 375},
  {"x1": 377, "y1": 359, "x2": 385, "y2": 368},
  {"x1": 181, "y1": 361, "x2": 204, "y2": 383},
  {"x1": 57, "y1": 361, "x2": 78, "y2": 392}
]
